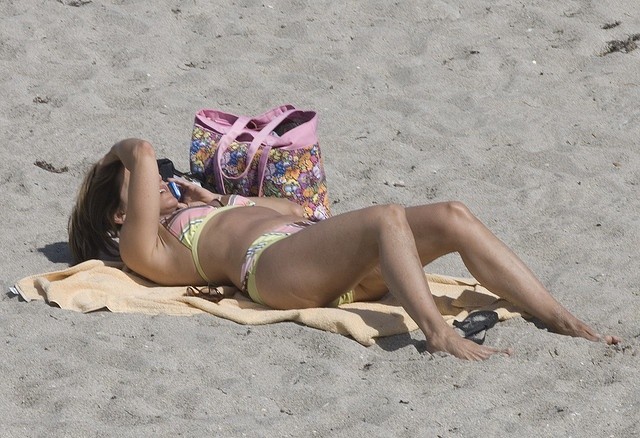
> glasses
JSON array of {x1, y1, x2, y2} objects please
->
[{"x1": 185, "y1": 280, "x2": 224, "y2": 304}]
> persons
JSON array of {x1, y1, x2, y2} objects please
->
[{"x1": 69, "y1": 137, "x2": 618, "y2": 362}]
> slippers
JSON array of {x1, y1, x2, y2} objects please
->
[{"x1": 451, "y1": 309, "x2": 498, "y2": 345}]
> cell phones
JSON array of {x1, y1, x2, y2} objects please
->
[{"x1": 167, "y1": 182, "x2": 182, "y2": 200}]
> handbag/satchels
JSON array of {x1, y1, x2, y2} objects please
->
[{"x1": 191, "y1": 104, "x2": 332, "y2": 223}]
